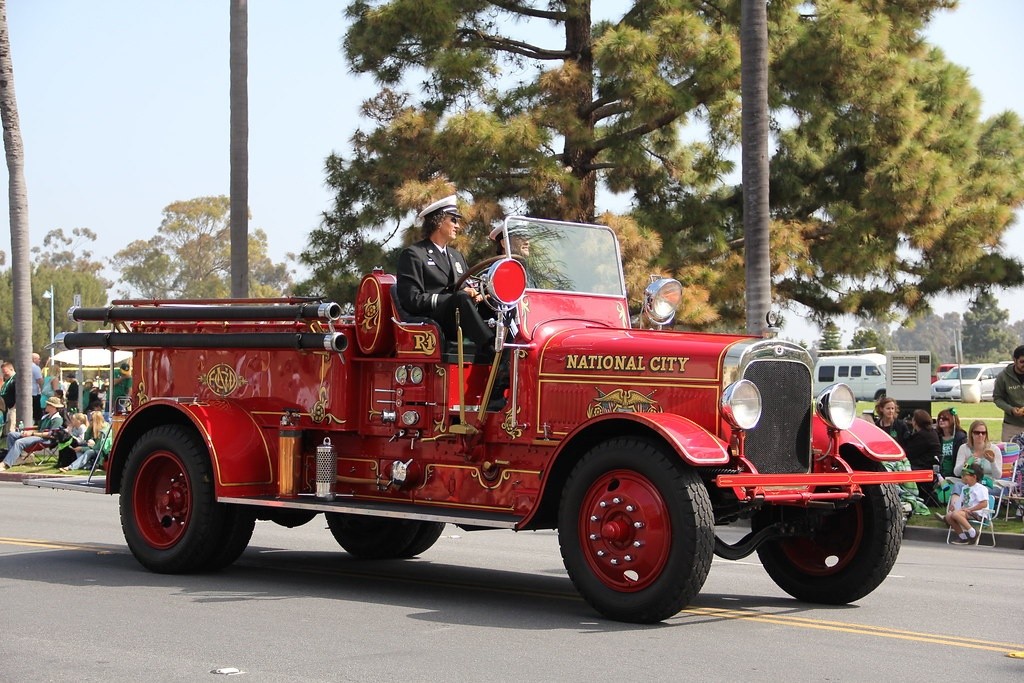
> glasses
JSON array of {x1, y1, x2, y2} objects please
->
[
  {"x1": 1016, "y1": 358, "x2": 1024, "y2": 363},
  {"x1": 441, "y1": 218, "x2": 457, "y2": 224},
  {"x1": 938, "y1": 417, "x2": 950, "y2": 421},
  {"x1": 971, "y1": 430, "x2": 987, "y2": 435}
]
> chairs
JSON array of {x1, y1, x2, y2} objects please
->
[
  {"x1": 16, "y1": 426, "x2": 60, "y2": 466},
  {"x1": 915, "y1": 434, "x2": 1024, "y2": 548}
]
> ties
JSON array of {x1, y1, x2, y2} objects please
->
[{"x1": 442, "y1": 251, "x2": 449, "y2": 263}]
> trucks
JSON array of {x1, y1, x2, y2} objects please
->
[{"x1": 55, "y1": 214, "x2": 935, "y2": 625}]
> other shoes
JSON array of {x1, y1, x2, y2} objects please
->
[
  {"x1": 0, "y1": 461, "x2": 7, "y2": 471},
  {"x1": 59, "y1": 467, "x2": 70, "y2": 472},
  {"x1": 935, "y1": 512, "x2": 950, "y2": 528},
  {"x1": 970, "y1": 529, "x2": 978, "y2": 545},
  {"x1": 951, "y1": 538, "x2": 969, "y2": 545}
]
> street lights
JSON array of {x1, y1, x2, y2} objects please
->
[{"x1": 41, "y1": 284, "x2": 55, "y2": 366}]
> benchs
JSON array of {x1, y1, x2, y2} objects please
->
[{"x1": 388, "y1": 284, "x2": 492, "y2": 365}]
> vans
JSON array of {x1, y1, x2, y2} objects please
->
[
  {"x1": 930, "y1": 361, "x2": 1015, "y2": 403},
  {"x1": 812, "y1": 352, "x2": 889, "y2": 401}
]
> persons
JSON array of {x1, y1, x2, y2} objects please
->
[
  {"x1": 876, "y1": 397, "x2": 1002, "y2": 521},
  {"x1": 0, "y1": 353, "x2": 132, "y2": 473},
  {"x1": 478, "y1": 217, "x2": 539, "y2": 320},
  {"x1": 992, "y1": 345, "x2": 1024, "y2": 442},
  {"x1": 396, "y1": 194, "x2": 513, "y2": 410},
  {"x1": 945, "y1": 463, "x2": 989, "y2": 545}
]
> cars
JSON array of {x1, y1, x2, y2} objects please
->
[{"x1": 930, "y1": 363, "x2": 956, "y2": 383}]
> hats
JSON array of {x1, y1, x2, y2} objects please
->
[
  {"x1": 85, "y1": 379, "x2": 93, "y2": 386},
  {"x1": 419, "y1": 194, "x2": 463, "y2": 218},
  {"x1": 120, "y1": 363, "x2": 130, "y2": 374},
  {"x1": 46, "y1": 396, "x2": 64, "y2": 408}
]
